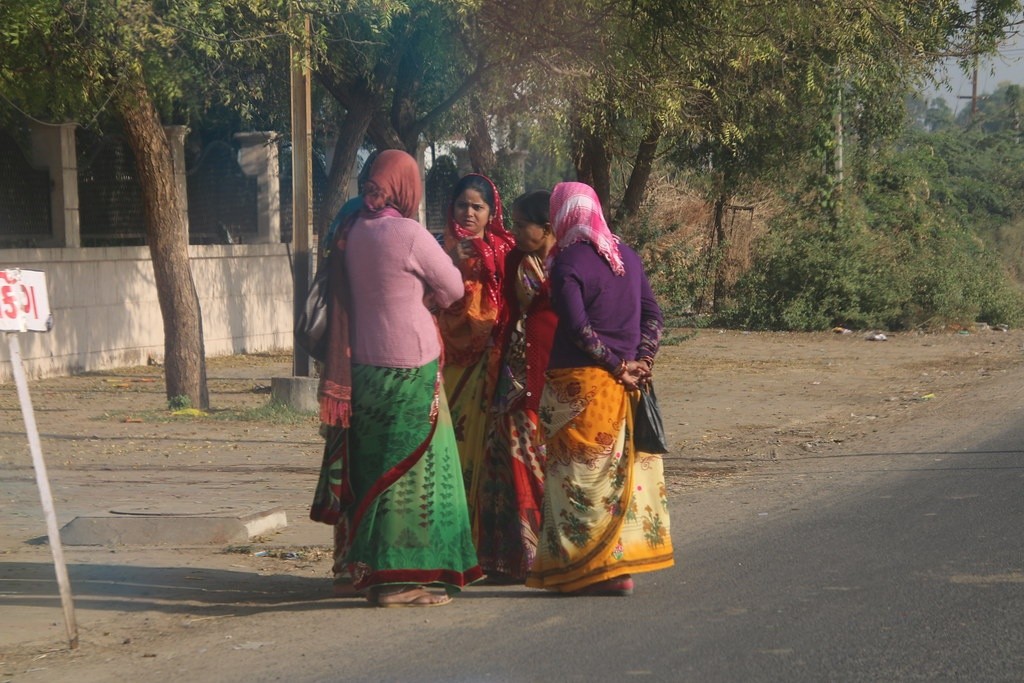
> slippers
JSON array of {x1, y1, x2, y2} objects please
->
[{"x1": 367, "y1": 593, "x2": 453, "y2": 608}]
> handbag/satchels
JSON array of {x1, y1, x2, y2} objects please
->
[
  {"x1": 293, "y1": 258, "x2": 332, "y2": 363},
  {"x1": 633, "y1": 382, "x2": 669, "y2": 453}
]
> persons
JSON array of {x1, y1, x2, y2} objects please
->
[
  {"x1": 309, "y1": 149, "x2": 484, "y2": 609},
  {"x1": 437, "y1": 173, "x2": 557, "y2": 584},
  {"x1": 523, "y1": 181, "x2": 675, "y2": 596}
]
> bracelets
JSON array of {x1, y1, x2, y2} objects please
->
[
  {"x1": 640, "y1": 356, "x2": 654, "y2": 369},
  {"x1": 614, "y1": 359, "x2": 628, "y2": 376}
]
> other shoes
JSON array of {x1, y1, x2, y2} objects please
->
[{"x1": 582, "y1": 574, "x2": 633, "y2": 596}]
